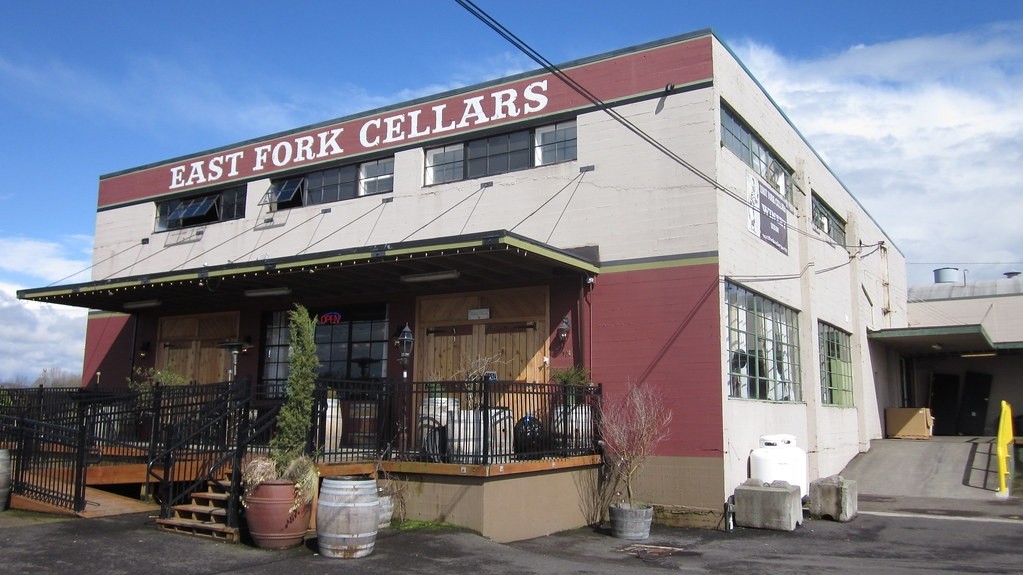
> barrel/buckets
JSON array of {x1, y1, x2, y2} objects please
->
[
  {"x1": 316, "y1": 475, "x2": 379, "y2": 558},
  {"x1": 749, "y1": 433, "x2": 808, "y2": 507}
]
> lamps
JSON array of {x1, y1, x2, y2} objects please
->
[
  {"x1": 397, "y1": 323, "x2": 414, "y2": 460},
  {"x1": 401, "y1": 270, "x2": 460, "y2": 283},
  {"x1": 242, "y1": 336, "x2": 253, "y2": 353},
  {"x1": 556, "y1": 319, "x2": 570, "y2": 345},
  {"x1": 245, "y1": 287, "x2": 293, "y2": 297},
  {"x1": 124, "y1": 299, "x2": 162, "y2": 309},
  {"x1": 139, "y1": 340, "x2": 151, "y2": 360}
]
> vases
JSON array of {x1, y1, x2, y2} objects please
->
[
  {"x1": 0, "y1": 448, "x2": 11, "y2": 511},
  {"x1": 317, "y1": 475, "x2": 379, "y2": 559}
]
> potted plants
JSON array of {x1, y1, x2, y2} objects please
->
[
  {"x1": 315, "y1": 386, "x2": 343, "y2": 453},
  {"x1": 548, "y1": 362, "x2": 596, "y2": 454},
  {"x1": 378, "y1": 461, "x2": 412, "y2": 529},
  {"x1": 596, "y1": 377, "x2": 674, "y2": 539},
  {"x1": 237, "y1": 303, "x2": 324, "y2": 548},
  {"x1": 421, "y1": 356, "x2": 515, "y2": 466},
  {"x1": 124, "y1": 364, "x2": 199, "y2": 448}
]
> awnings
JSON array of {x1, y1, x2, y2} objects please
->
[{"x1": 17, "y1": 229, "x2": 598, "y2": 319}]
